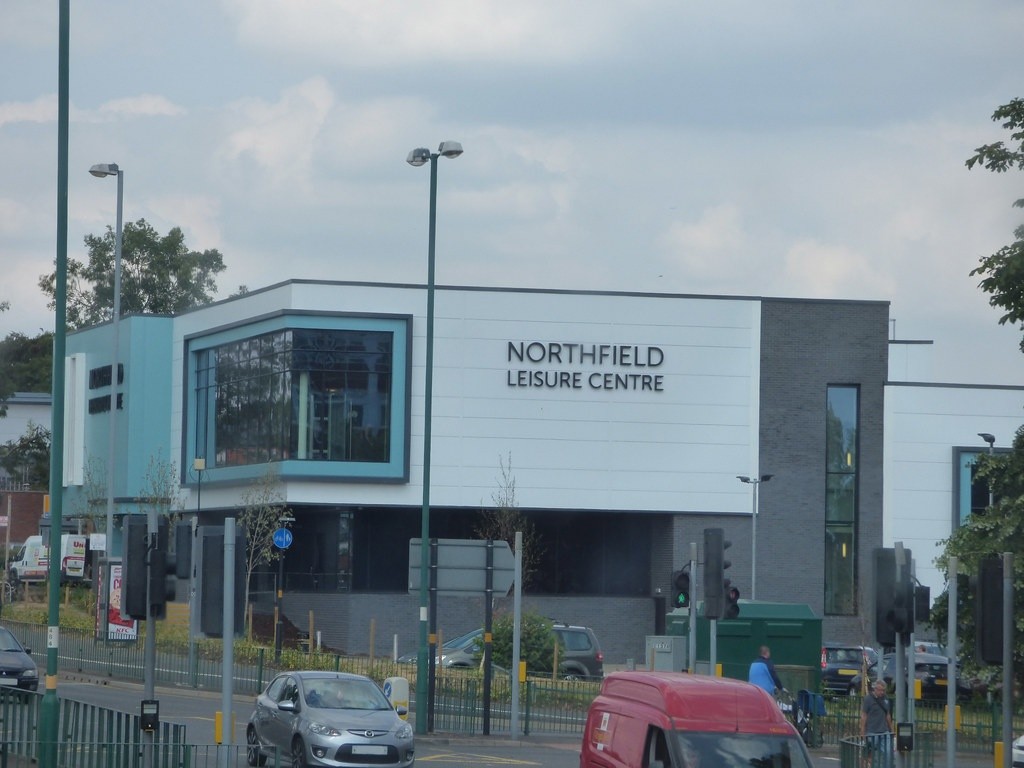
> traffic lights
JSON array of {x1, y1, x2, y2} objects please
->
[
  {"x1": 670, "y1": 570, "x2": 690, "y2": 608},
  {"x1": 724, "y1": 586, "x2": 740, "y2": 618},
  {"x1": 704, "y1": 529, "x2": 731, "y2": 619}
]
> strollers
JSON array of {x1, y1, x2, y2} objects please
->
[{"x1": 771, "y1": 686, "x2": 825, "y2": 747}]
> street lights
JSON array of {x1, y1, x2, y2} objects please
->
[
  {"x1": 979, "y1": 433, "x2": 996, "y2": 510},
  {"x1": 737, "y1": 475, "x2": 776, "y2": 599},
  {"x1": 274, "y1": 518, "x2": 297, "y2": 662},
  {"x1": 87, "y1": 162, "x2": 123, "y2": 651},
  {"x1": 407, "y1": 140, "x2": 464, "y2": 736}
]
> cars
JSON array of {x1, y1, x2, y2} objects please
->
[
  {"x1": 0, "y1": 626, "x2": 40, "y2": 701},
  {"x1": 846, "y1": 652, "x2": 972, "y2": 705},
  {"x1": 246, "y1": 670, "x2": 415, "y2": 768}
]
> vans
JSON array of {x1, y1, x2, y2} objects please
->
[
  {"x1": 579, "y1": 672, "x2": 813, "y2": 768},
  {"x1": 7, "y1": 534, "x2": 93, "y2": 591},
  {"x1": 820, "y1": 644, "x2": 880, "y2": 694},
  {"x1": 398, "y1": 624, "x2": 604, "y2": 683}
]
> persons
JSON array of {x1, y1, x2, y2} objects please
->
[
  {"x1": 916, "y1": 644, "x2": 928, "y2": 653},
  {"x1": 857, "y1": 679, "x2": 897, "y2": 767},
  {"x1": 682, "y1": 745, "x2": 701, "y2": 768},
  {"x1": 747, "y1": 644, "x2": 784, "y2": 699}
]
